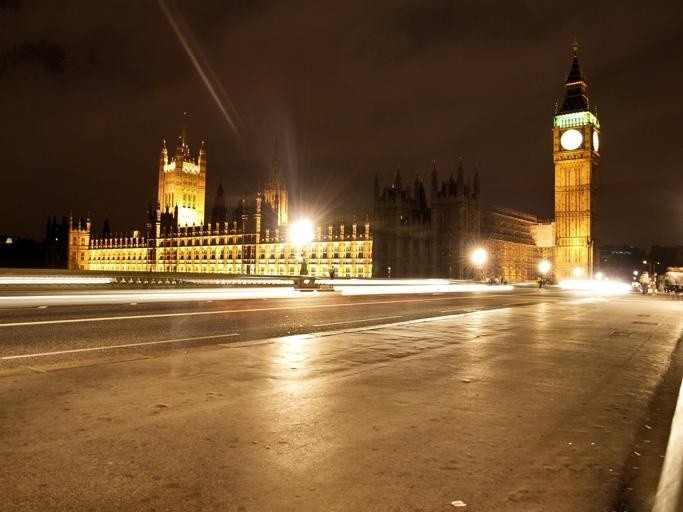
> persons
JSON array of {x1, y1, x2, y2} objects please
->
[{"x1": 638, "y1": 270, "x2": 682, "y2": 294}]
